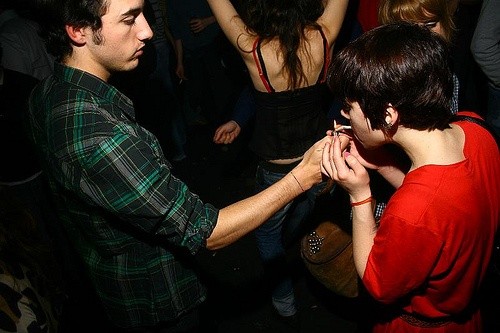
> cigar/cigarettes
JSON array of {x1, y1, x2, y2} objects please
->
[
  {"x1": 335, "y1": 125, "x2": 353, "y2": 130},
  {"x1": 179, "y1": 78, "x2": 183, "y2": 86}
]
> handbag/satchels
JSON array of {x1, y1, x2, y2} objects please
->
[{"x1": 300, "y1": 221, "x2": 359, "y2": 298}]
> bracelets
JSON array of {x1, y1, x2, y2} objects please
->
[{"x1": 350, "y1": 195, "x2": 377, "y2": 213}]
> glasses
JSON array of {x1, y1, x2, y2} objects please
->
[{"x1": 425, "y1": 19, "x2": 440, "y2": 30}]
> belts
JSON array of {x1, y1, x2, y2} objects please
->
[{"x1": 259, "y1": 160, "x2": 303, "y2": 174}]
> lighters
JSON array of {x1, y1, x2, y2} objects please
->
[{"x1": 331, "y1": 119, "x2": 338, "y2": 136}]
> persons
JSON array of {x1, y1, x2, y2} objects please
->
[
  {"x1": 141, "y1": 1, "x2": 176, "y2": 97},
  {"x1": 378, "y1": 0, "x2": 492, "y2": 117},
  {"x1": 164, "y1": 0, "x2": 218, "y2": 128},
  {"x1": 28, "y1": 0, "x2": 353, "y2": 333},
  {"x1": 321, "y1": 22, "x2": 500, "y2": 333},
  {"x1": 205, "y1": 0, "x2": 355, "y2": 330},
  {"x1": 470, "y1": 0, "x2": 500, "y2": 144}
]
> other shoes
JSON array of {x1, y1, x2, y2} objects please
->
[
  {"x1": 187, "y1": 117, "x2": 207, "y2": 125},
  {"x1": 272, "y1": 289, "x2": 297, "y2": 317}
]
